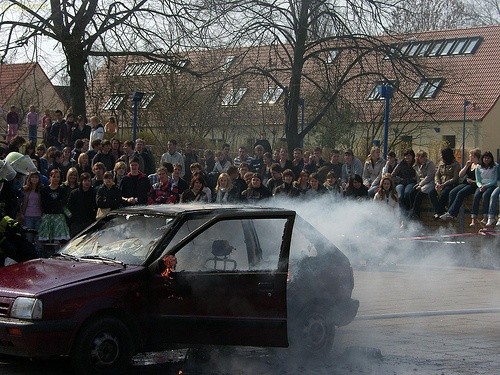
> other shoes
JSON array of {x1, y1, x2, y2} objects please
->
[
  {"x1": 486, "y1": 219, "x2": 495, "y2": 226},
  {"x1": 496, "y1": 219, "x2": 500, "y2": 226},
  {"x1": 439, "y1": 212, "x2": 450, "y2": 222},
  {"x1": 480, "y1": 219, "x2": 486, "y2": 225},
  {"x1": 470, "y1": 223, "x2": 476, "y2": 226}
]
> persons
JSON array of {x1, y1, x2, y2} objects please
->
[
  {"x1": 43, "y1": 120, "x2": 53, "y2": 148},
  {"x1": 274, "y1": 170, "x2": 298, "y2": 197},
  {"x1": 343, "y1": 149, "x2": 363, "y2": 186},
  {"x1": 210, "y1": 174, "x2": 234, "y2": 203},
  {"x1": 50, "y1": 109, "x2": 66, "y2": 146},
  {"x1": 42, "y1": 109, "x2": 52, "y2": 139},
  {"x1": 0, "y1": 134, "x2": 343, "y2": 266},
  {"x1": 104, "y1": 117, "x2": 117, "y2": 133},
  {"x1": 343, "y1": 175, "x2": 368, "y2": 197},
  {"x1": 294, "y1": 170, "x2": 310, "y2": 193},
  {"x1": 58, "y1": 113, "x2": 76, "y2": 147},
  {"x1": 88, "y1": 116, "x2": 103, "y2": 150},
  {"x1": 6, "y1": 105, "x2": 19, "y2": 141},
  {"x1": 307, "y1": 174, "x2": 326, "y2": 195},
  {"x1": 322, "y1": 172, "x2": 340, "y2": 193},
  {"x1": 364, "y1": 146, "x2": 500, "y2": 225},
  {"x1": 240, "y1": 173, "x2": 271, "y2": 201},
  {"x1": 26, "y1": 105, "x2": 38, "y2": 145},
  {"x1": 71, "y1": 118, "x2": 91, "y2": 145}
]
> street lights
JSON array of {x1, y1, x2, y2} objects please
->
[
  {"x1": 133, "y1": 91, "x2": 144, "y2": 143},
  {"x1": 380, "y1": 85, "x2": 393, "y2": 160},
  {"x1": 462, "y1": 99, "x2": 472, "y2": 170},
  {"x1": 298, "y1": 98, "x2": 305, "y2": 147}
]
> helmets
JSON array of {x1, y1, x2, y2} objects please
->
[
  {"x1": 4, "y1": 152, "x2": 37, "y2": 175},
  {"x1": 0, "y1": 160, "x2": 16, "y2": 181}
]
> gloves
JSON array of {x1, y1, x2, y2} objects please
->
[{"x1": 19, "y1": 225, "x2": 39, "y2": 235}]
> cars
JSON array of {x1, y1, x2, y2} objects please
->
[{"x1": 0, "y1": 202, "x2": 362, "y2": 375}]
